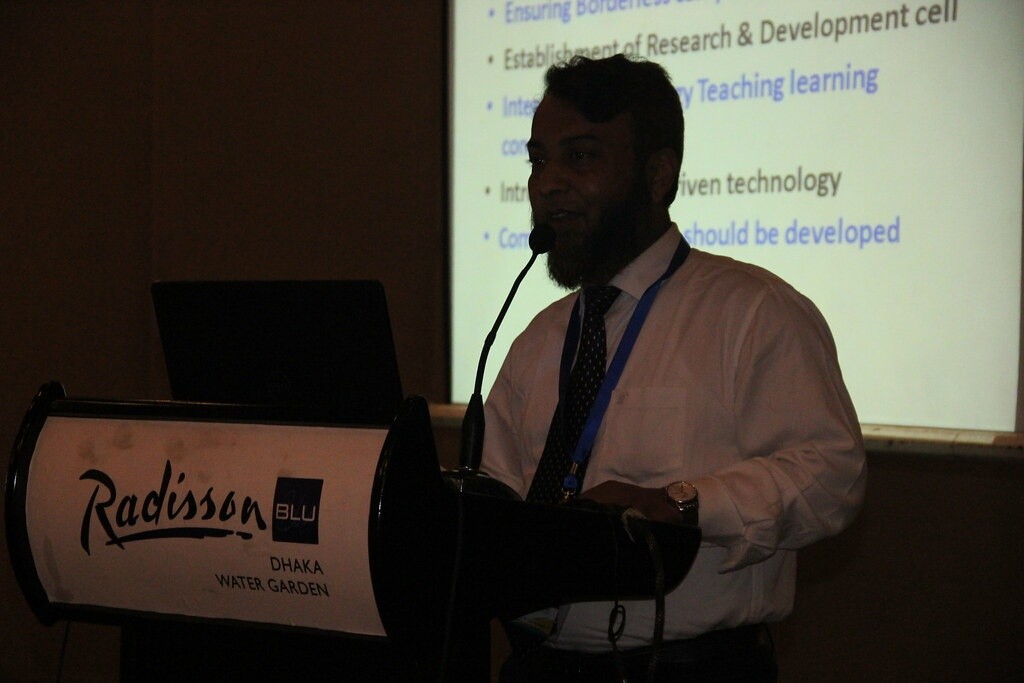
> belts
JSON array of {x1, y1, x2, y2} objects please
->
[{"x1": 512, "y1": 624, "x2": 766, "y2": 671}]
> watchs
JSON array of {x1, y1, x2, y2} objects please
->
[{"x1": 663, "y1": 482, "x2": 700, "y2": 525}]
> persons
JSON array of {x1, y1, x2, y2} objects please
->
[{"x1": 447, "y1": 54, "x2": 864, "y2": 682}]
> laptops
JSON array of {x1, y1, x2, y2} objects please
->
[{"x1": 151, "y1": 278, "x2": 403, "y2": 425}]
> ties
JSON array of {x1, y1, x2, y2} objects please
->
[{"x1": 507, "y1": 284, "x2": 621, "y2": 661}]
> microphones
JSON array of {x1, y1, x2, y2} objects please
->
[{"x1": 442, "y1": 223, "x2": 557, "y2": 501}]
